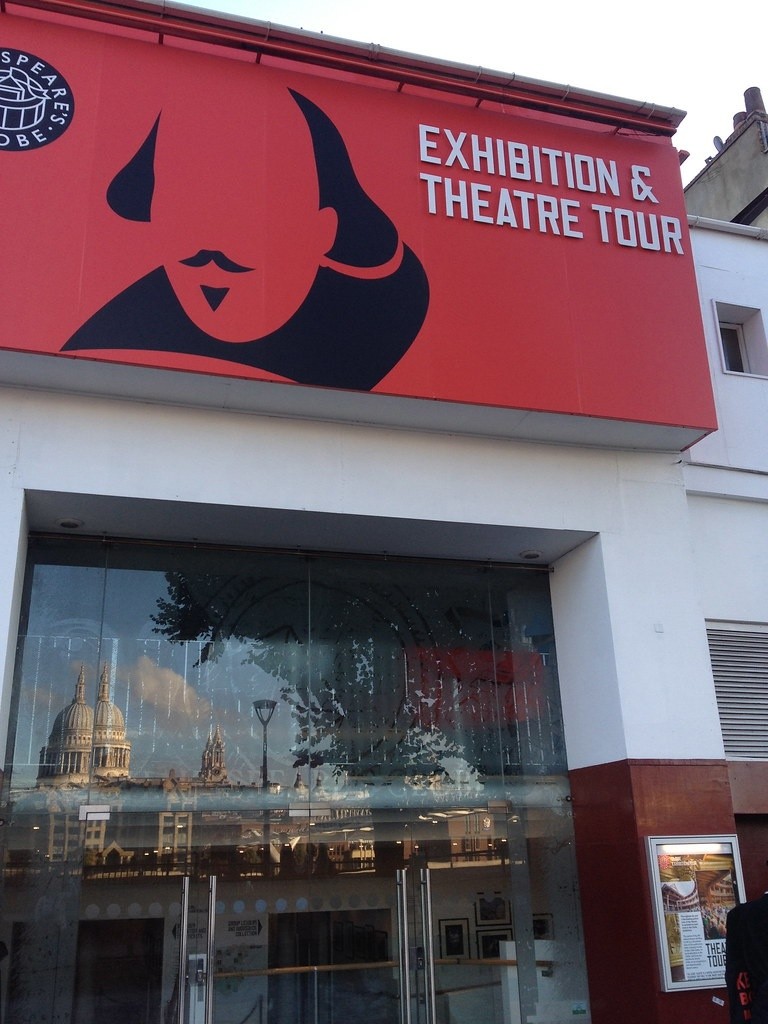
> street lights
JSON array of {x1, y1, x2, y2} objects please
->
[{"x1": 251, "y1": 696, "x2": 279, "y2": 874}]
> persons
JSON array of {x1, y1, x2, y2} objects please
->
[{"x1": 725, "y1": 890, "x2": 768, "y2": 1024}]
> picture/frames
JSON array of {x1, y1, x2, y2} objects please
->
[
  {"x1": 475, "y1": 891, "x2": 512, "y2": 926},
  {"x1": 533, "y1": 913, "x2": 554, "y2": 939},
  {"x1": 475, "y1": 928, "x2": 514, "y2": 959},
  {"x1": 439, "y1": 918, "x2": 471, "y2": 960}
]
